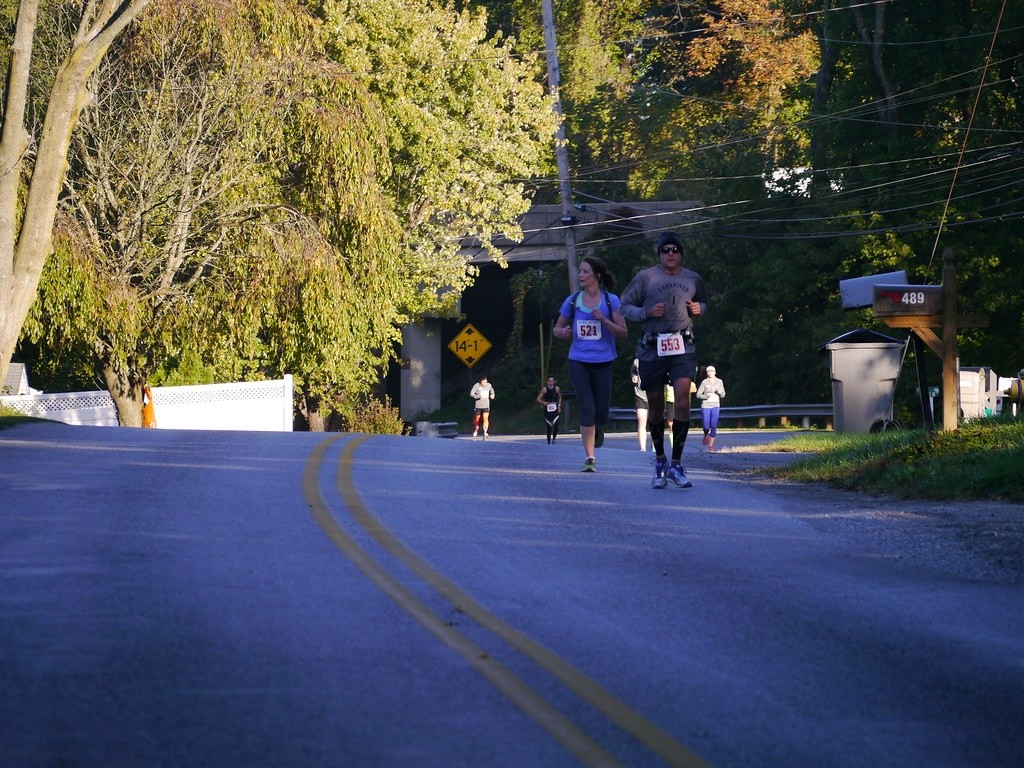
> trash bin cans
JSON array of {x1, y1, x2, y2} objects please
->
[{"x1": 818, "y1": 328, "x2": 906, "y2": 434}]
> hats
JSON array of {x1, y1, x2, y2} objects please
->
[
  {"x1": 658, "y1": 232, "x2": 683, "y2": 258},
  {"x1": 706, "y1": 366, "x2": 716, "y2": 372}
]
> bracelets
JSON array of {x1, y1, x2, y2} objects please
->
[{"x1": 558, "y1": 404, "x2": 561, "y2": 407}]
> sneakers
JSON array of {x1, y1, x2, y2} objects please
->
[
  {"x1": 594, "y1": 426, "x2": 604, "y2": 449},
  {"x1": 652, "y1": 460, "x2": 669, "y2": 488},
  {"x1": 666, "y1": 465, "x2": 692, "y2": 488},
  {"x1": 580, "y1": 457, "x2": 596, "y2": 472}
]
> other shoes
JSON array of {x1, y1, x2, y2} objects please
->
[
  {"x1": 703, "y1": 435, "x2": 708, "y2": 445},
  {"x1": 473, "y1": 430, "x2": 479, "y2": 437},
  {"x1": 552, "y1": 440, "x2": 555, "y2": 445},
  {"x1": 483, "y1": 433, "x2": 489, "y2": 438},
  {"x1": 707, "y1": 445, "x2": 715, "y2": 451}
]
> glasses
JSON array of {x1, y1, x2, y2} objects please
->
[{"x1": 659, "y1": 247, "x2": 680, "y2": 254}]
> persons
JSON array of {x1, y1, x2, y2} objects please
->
[
  {"x1": 470, "y1": 376, "x2": 495, "y2": 439},
  {"x1": 631, "y1": 359, "x2": 697, "y2": 452},
  {"x1": 619, "y1": 232, "x2": 708, "y2": 489},
  {"x1": 696, "y1": 366, "x2": 726, "y2": 452},
  {"x1": 536, "y1": 376, "x2": 562, "y2": 445},
  {"x1": 553, "y1": 256, "x2": 628, "y2": 472}
]
